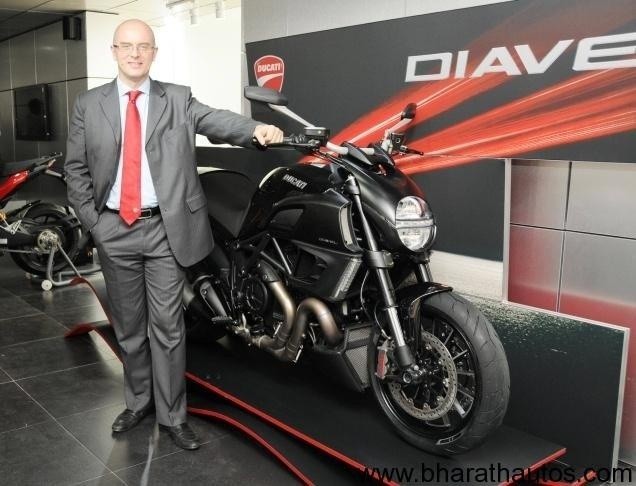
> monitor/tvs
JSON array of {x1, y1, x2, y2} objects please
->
[{"x1": 14, "y1": 84, "x2": 51, "y2": 142}]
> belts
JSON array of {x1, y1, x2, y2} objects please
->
[{"x1": 104, "y1": 206, "x2": 161, "y2": 219}]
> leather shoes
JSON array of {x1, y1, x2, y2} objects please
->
[
  {"x1": 158, "y1": 422, "x2": 201, "y2": 452},
  {"x1": 111, "y1": 401, "x2": 153, "y2": 435}
]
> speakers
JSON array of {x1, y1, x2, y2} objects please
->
[{"x1": 62, "y1": 16, "x2": 81, "y2": 40}]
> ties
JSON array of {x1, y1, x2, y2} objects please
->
[{"x1": 117, "y1": 89, "x2": 144, "y2": 227}]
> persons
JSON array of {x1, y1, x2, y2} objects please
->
[{"x1": 62, "y1": 18, "x2": 286, "y2": 452}]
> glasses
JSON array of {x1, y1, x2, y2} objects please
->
[{"x1": 112, "y1": 44, "x2": 157, "y2": 55}]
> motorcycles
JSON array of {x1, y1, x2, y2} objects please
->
[
  {"x1": 0, "y1": 151, "x2": 83, "y2": 276},
  {"x1": 182, "y1": 85, "x2": 512, "y2": 456}
]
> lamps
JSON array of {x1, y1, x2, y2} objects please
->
[
  {"x1": 189, "y1": 0, "x2": 200, "y2": 24},
  {"x1": 215, "y1": 0, "x2": 225, "y2": 19}
]
